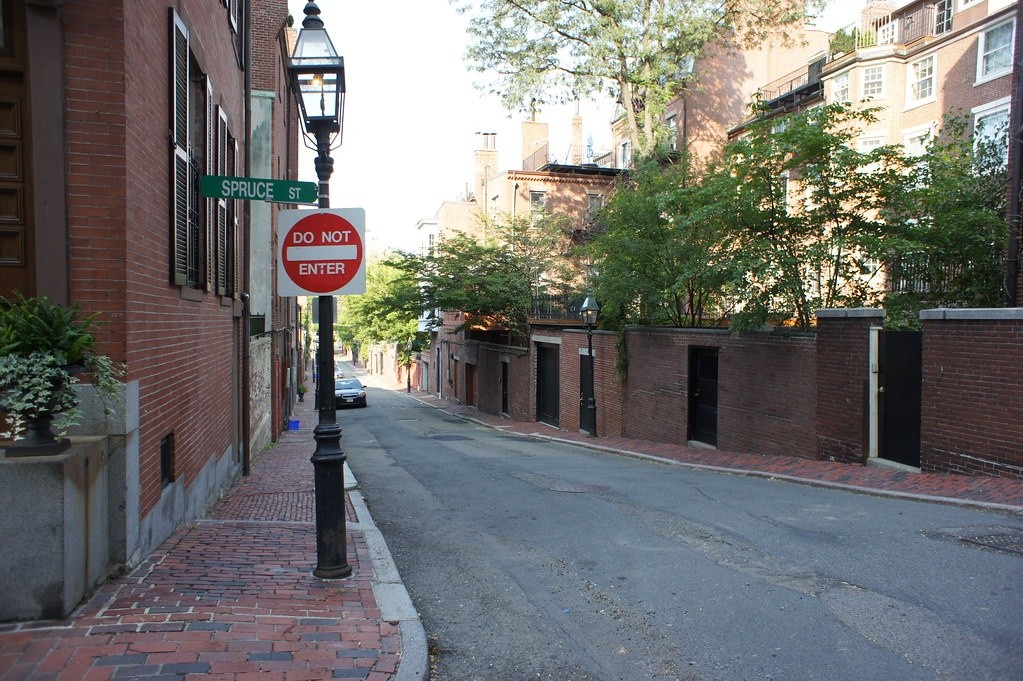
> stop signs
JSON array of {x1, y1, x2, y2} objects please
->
[{"x1": 276, "y1": 207, "x2": 368, "y2": 298}]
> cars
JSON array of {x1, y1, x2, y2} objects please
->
[
  {"x1": 335, "y1": 379, "x2": 367, "y2": 408},
  {"x1": 334, "y1": 367, "x2": 344, "y2": 379}
]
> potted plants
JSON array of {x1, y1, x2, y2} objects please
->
[
  {"x1": 297, "y1": 384, "x2": 308, "y2": 402},
  {"x1": 0, "y1": 289, "x2": 128, "y2": 457},
  {"x1": 830, "y1": 28, "x2": 849, "y2": 60}
]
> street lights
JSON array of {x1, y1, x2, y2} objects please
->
[
  {"x1": 287, "y1": 3, "x2": 369, "y2": 583},
  {"x1": 406, "y1": 340, "x2": 413, "y2": 393},
  {"x1": 580, "y1": 297, "x2": 601, "y2": 438}
]
